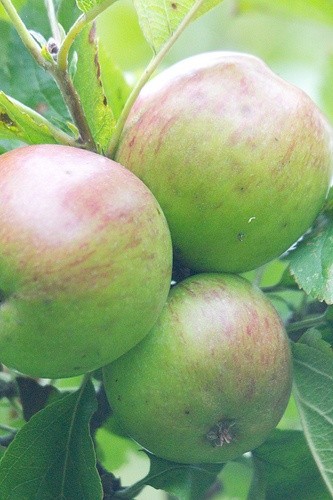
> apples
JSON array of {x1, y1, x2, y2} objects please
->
[{"x1": 0, "y1": 51, "x2": 333, "y2": 462}]
